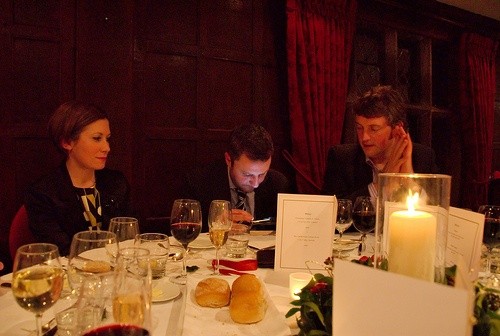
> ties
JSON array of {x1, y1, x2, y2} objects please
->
[{"x1": 236, "y1": 188, "x2": 247, "y2": 211}]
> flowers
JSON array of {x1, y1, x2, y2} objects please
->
[{"x1": 286, "y1": 253, "x2": 500, "y2": 336}]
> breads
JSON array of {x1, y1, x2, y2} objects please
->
[
  {"x1": 86, "y1": 260, "x2": 112, "y2": 272},
  {"x1": 230, "y1": 292, "x2": 267, "y2": 323},
  {"x1": 231, "y1": 274, "x2": 264, "y2": 295},
  {"x1": 194, "y1": 277, "x2": 230, "y2": 307}
]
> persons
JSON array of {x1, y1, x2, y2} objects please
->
[
  {"x1": 28, "y1": 101, "x2": 128, "y2": 258},
  {"x1": 323, "y1": 85, "x2": 437, "y2": 205},
  {"x1": 193, "y1": 122, "x2": 292, "y2": 230}
]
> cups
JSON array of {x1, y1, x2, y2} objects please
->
[
  {"x1": 375, "y1": 172, "x2": 451, "y2": 286},
  {"x1": 51, "y1": 273, "x2": 104, "y2": 336},
  {"x1": 106, "y1": 217, "x2": 138, "y2": 269},
  {"x1": 134, "y1": 232, "x2": 170, "y2": 279},
  {"x1": 289, "y1": 272, "x2": 314, "y2": 307},
  {"x1": 75, "y1": 271, "x2": 153, "y2": 336},
  {"x1": 227, "y1": 213, "x2": 251, "y2": 258},
  {"x1": 66, "y1": 231, "x2": 124, "y2": 322},
  {"x1": 115, "y1": 248, "x2": 151, "y2": 279}
]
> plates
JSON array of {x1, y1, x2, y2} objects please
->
[
  {"x1": 169, "y1": 232, "x2": 217, "y2": 249},
  {"x1": 332, "y1": 234, "x2": 359, "y2": 250},
  {"x1": 150, "y1": 276, "x2": 181, "y2": 302}
]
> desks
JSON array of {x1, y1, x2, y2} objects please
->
[{"x1": 0, "y1": 231, "x2": 500, "y2": 336}]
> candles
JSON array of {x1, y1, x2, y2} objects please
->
[{"x1": 386, "y1": 189, "x2": 435, "y2": 282}]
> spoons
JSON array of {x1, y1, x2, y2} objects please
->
[{"x1": 171, "y1": 251, "x2": 184, "y2": 261}]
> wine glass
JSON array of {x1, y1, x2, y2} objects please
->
[
  {"x1": 207, "y1": 200, "x2": 231, "y2": 278},
  {"x1": 332, "y1": 199, "x2": 354, "y2": 259},
  {"x1": 353, "y1": 195, "x2": 377, "y2": 259},
  {"x1": 169, "y1": 199, "x2": 202, "y2": 284},
  {"x1": 12, "y1": 244, "x2": 63, "y2": 336},
  {"x1": 476, "y1": 205, "x2": 500, "y2": 288}
]
